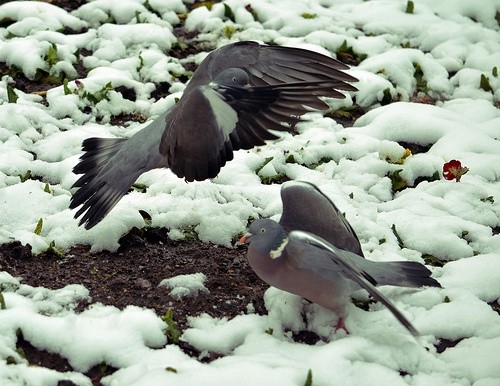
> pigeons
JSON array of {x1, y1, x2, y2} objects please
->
[
  {"x1": 239, "y1": 180, "x2": 442, "y2": 343},
  {"x1": 69, "y1": 40, "x2": 360, "y2": 231}
]
